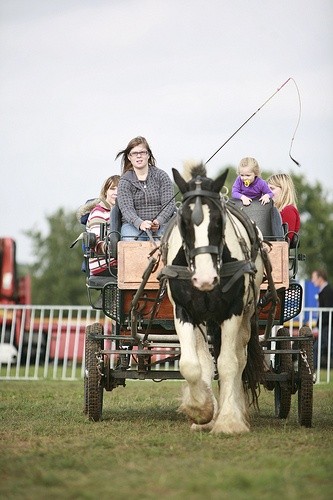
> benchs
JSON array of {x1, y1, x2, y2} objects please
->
[
  {"x1": 278, "y1": 227, "x2": 303, "y2": 288},
  {"x1": 82, "y1": 227, "x2": 118, "y2": 293},
  {"x1": 108, "y1": 229, "x2": 292, "y2": 329}
]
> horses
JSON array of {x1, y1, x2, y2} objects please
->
[{"x1": 161, "y1": 161, "x2": 268, "y2": 436}]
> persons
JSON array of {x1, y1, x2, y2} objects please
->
[
  {"x1": 114, "y1": 137, "x2": 175, "y2": 241},
  {"x1": 231, "y1": 157, "x2": 273, "y2": 206},
  {"x1": 266, "y1": 173, "x2": 300, "y2": 246},
  {"x1": 86, "y1": 175, "x2": 121, "y2": 276},
  {"x1": 80, "y1": 198, "x2": 100, "y2": 272},
  {"x1": 311, "y1": 269, "x2": 333, "y2": 383}
]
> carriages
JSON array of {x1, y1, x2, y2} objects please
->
[{"x1": 85, "y1": 163, "x2": 315, "y2": 434}]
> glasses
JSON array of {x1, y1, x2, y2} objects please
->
[{"x1": 129, "y1": 150, "x2": 149, "y2": 157}]
